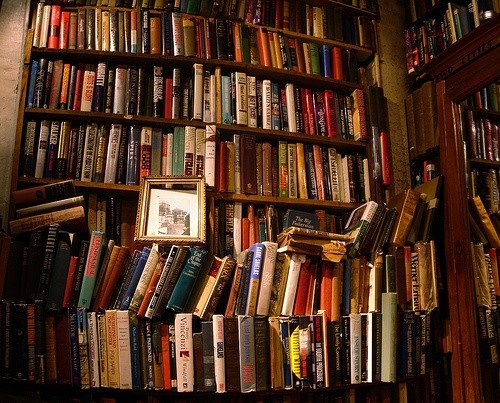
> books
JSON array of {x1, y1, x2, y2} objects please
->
[{"x1": 0, "y1": 0, "x2": 500, "y2": 393}]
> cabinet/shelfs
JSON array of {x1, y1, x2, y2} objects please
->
[
  {"x1": 0, "y1": 0, "x2": 406, "y2": 403},
  {"x1": 414, "y1": 20, "x2": 500, "y2": 403}
]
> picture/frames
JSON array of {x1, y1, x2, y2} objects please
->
[{"x1": 132, "y1": 174, "x2": 212, "y2": 246}]
